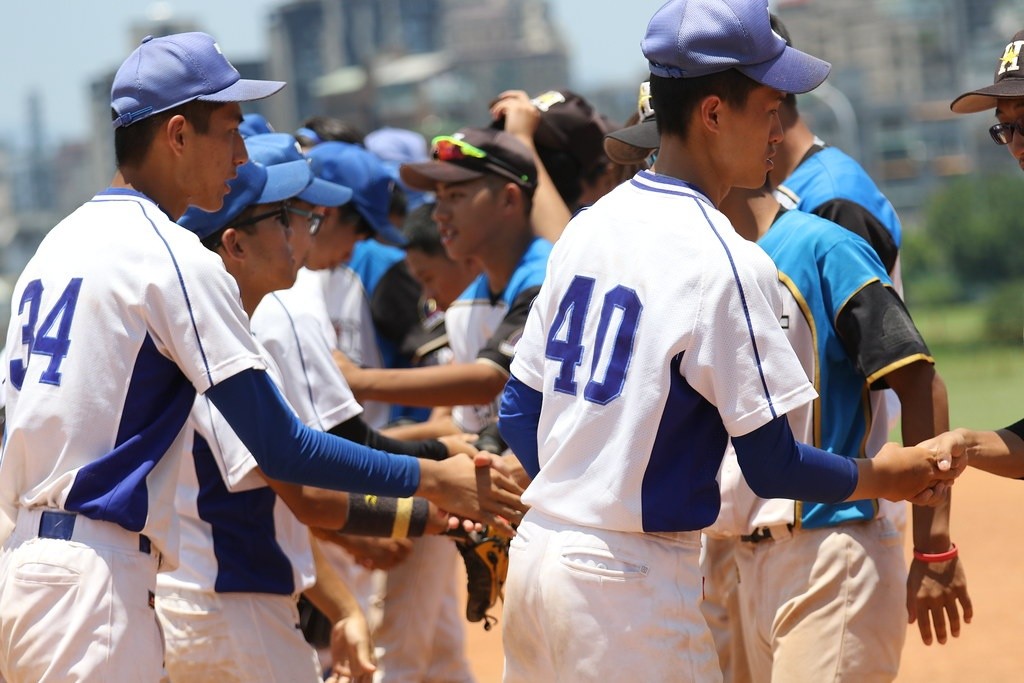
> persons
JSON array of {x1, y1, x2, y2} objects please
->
[
  {"x1": 0, "y1": 0, "x2": 1024, "y2": 683},
  {"x1": 494, "y1": 0, "x2": 960, "y2": 683},
  {"x1": 0, "y1": 30, "x2": 531, "y2": 683}
]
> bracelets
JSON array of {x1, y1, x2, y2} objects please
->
[{"x1": 912, "y1": 543, "x2": 957, "y2": 562}]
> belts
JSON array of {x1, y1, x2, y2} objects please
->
[
  {"x1": 741, "y1": 522, "x2": 793, "y2": 543},
  {"x1": 39, "y1": 511, "x2": 162, "y2": 572}
]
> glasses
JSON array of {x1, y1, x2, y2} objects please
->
[
  {"x1": 429, "y1": 136, "x2": 530, "y2": 191},
  {"x1": 209, "y1": 208, "x2": 291, "y2": 245},
  {"x1": 989, "y1": 116, "x2": 1024, "y2": 145},
  {"x1": 287, "y1": 204, "x2": 323, "y2": 235}
]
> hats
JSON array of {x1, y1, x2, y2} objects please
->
[
  {"x1": 640, "y1": 0, "x2": 831, "y2": 93},
  {"x1": 176, "y1": 112, "x2": 430, "y2": 241},
  {"x1": 110, "y1": 32, "x2": 285, "y2": 131},
  {"x1": 400, "y1": 130, "x2": 537, "y2": 203},
  {"x1": 532, "y1": 91, "x2": 607, "y2": 183},
  {"x1": 609, "y1": 82, "x2": 666, "y2": 149},
  {"x1": 948, "y1": 31, "x2": 1024, "y2": 115}
]
